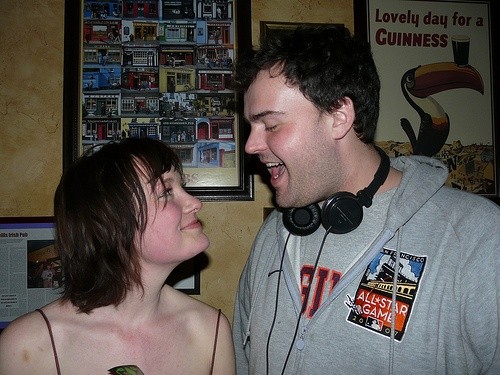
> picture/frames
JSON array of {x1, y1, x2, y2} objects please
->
[
  {"x1": 62, "y1": 0, "x2": 255, "y2": 202},
  {"x1": 353, "y1": 0, "x2": 500, "y2": 206}
]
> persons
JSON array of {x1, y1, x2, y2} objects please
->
[
  {"x1": 231, "y1": 27, "x2": 500, "y2": 375},
  {"x1": 32, "y1": 260, "x2": 62, "y2": 288},
  {"x1": 0, "y1": 135, "x2": 235, "y2": 374}
]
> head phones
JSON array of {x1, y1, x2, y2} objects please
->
[{"x1": 281, "y1": 145, "x2": 391, "y2": 237}]
